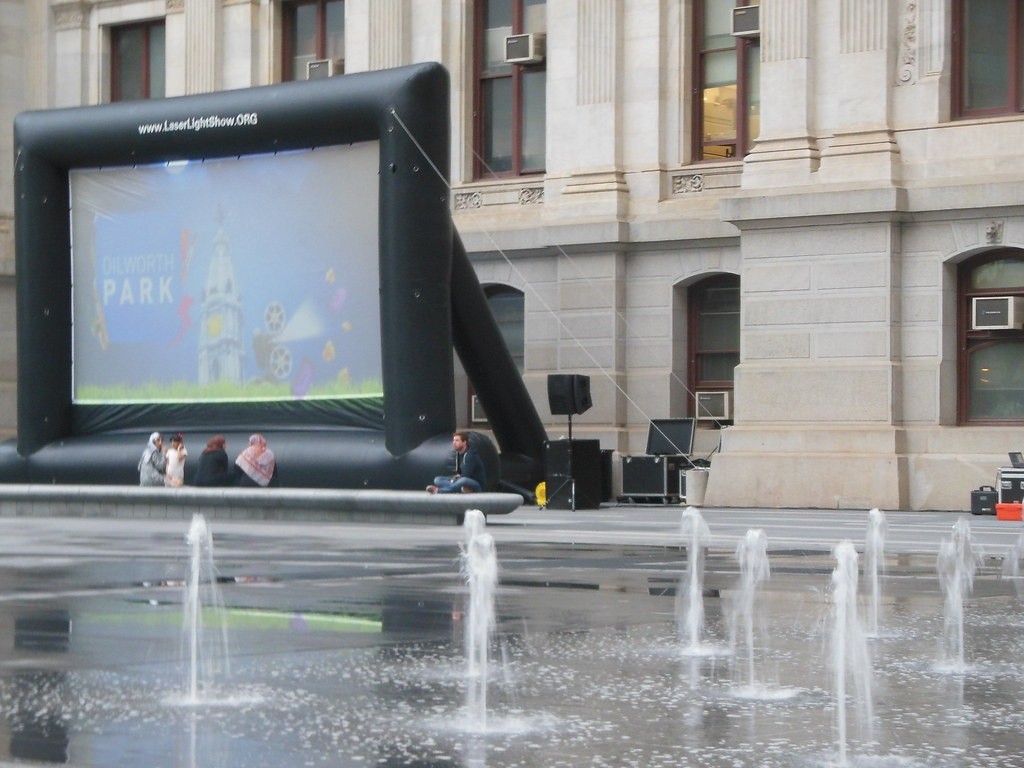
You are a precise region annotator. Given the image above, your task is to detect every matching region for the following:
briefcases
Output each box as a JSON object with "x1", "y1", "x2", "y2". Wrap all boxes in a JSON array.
[{"x1": 971, "y1": 486, "x2": 998, "y2": 515}]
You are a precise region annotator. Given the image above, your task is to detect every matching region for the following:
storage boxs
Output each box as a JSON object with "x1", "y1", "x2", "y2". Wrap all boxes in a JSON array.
[
  {"x1": 619, "y1": 454, "x2": 712, "y2": 505},
  {"x1": 995, "y1": 466, "x2": 1024, "y2": 519}
]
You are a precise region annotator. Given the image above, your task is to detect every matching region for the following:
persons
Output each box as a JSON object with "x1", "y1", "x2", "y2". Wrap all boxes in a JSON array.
[
  {"x1": 234, "y1": 435, "x2": 278, "y2": 488},
  {"x1": 137, "y1": 433, "x2": 188, "y2": 488},
  {"x1": 193, "y1": 435, "x2": 230, "y2": 487},
  {"x1": 426, "y1": 432, "x2": 486, "y2": 494}
]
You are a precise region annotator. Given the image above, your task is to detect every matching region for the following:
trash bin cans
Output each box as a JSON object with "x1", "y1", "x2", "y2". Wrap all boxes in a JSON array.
[{"x1": 685, "y1": 469, "x2": 709, "y2": 507}]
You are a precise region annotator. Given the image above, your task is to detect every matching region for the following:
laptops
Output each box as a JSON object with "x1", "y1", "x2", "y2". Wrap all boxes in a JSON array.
[{"x1": 1009, "y1": 452, "x2": 1024, "y2": 468}]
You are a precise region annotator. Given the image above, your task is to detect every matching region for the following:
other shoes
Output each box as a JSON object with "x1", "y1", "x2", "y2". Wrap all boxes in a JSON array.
[
  {"x1": 426, "y1": 485, "x2": 438, "y2": 494},
  {"x1": 461, "y1": 486, "x2": 473, "y2": 493}
]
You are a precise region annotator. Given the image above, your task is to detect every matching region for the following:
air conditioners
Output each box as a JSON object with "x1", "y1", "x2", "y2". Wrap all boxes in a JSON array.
[
  {"x1": 695, "y1": 391, "x2": 734, "y2": 420},
  {"x1": 971, "y1": 296, "x2": 1024, "y2": 330},
  {"x1": 306, "y1": 58, "x2": 344, "y2": 81},
  {"x1": 504, "y1": 33, "x2": 546, "y2": 65},
  {"x1": 471, "y1": 394, "x2": 490, "y2": 424},
  {"x1": 730, "y1": 5, "x2": 761, "y2": 39}
]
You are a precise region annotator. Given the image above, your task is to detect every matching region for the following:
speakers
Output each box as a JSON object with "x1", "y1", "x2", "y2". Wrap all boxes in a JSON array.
[
  {"x1": 548, "y1": 374, "x2": 593, "y2": 415},
  {"x1": 543, "y1": 438, "x2": 602, "y2": 509}
]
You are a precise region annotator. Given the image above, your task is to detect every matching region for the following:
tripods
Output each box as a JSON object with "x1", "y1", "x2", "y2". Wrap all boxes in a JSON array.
[{"x1": 540, "y1": 415, "x2": 600, "y2": 513}]
